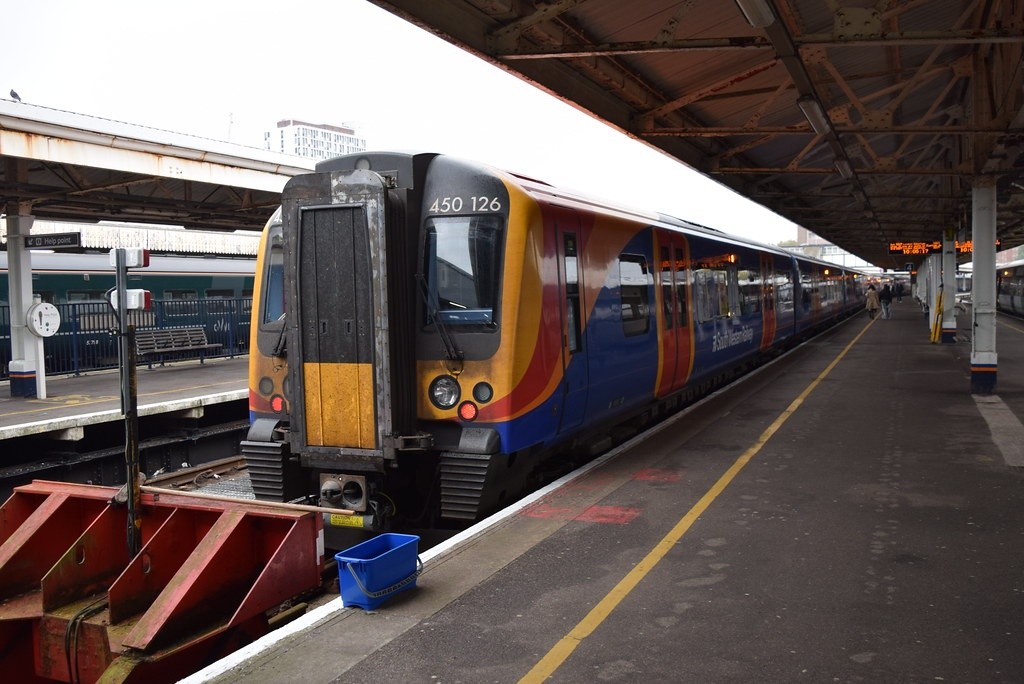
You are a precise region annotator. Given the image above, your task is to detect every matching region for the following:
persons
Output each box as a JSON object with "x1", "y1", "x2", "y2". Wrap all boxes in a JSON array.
[
  {"x1": 801, "y1": 287, "x2": 822, "y2": 312},
  {"x1": 864, "y1": 285, "x2": 880, "y2": 320},
  {"x1": 878, "y1": 284, "x2": 904, "y2": 321}
]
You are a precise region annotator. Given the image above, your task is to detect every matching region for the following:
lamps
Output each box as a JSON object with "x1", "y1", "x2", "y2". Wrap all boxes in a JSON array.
[
  {"x1": 797, "y1": 94, "x2": 832, "y2": 136},
  {"x1": 735, "y1": 0, "x2": 776, "y2": 29},
  {"x1": 853, "y1": 189, "x2": 888, "y2": 246},
  {"x1": 833, "y1": 156, "x2": 854, "y2": 179}
]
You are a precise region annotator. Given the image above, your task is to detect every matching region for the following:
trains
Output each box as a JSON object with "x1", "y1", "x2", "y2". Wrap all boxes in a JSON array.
[
  {"x1": 241, "y1": 149, "x2": 892, "y2": 528},
  {"x1": 956, "y1": 259, "x2": 1024, "y2": 318},
  {"x1": 0, "y1": 254, "x2": 256, "y2": 381}
]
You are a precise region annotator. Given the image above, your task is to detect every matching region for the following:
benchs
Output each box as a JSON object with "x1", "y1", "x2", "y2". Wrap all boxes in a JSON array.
[{"x1": 134, "y1": 328, "x2": 223, "y2": 371}]
